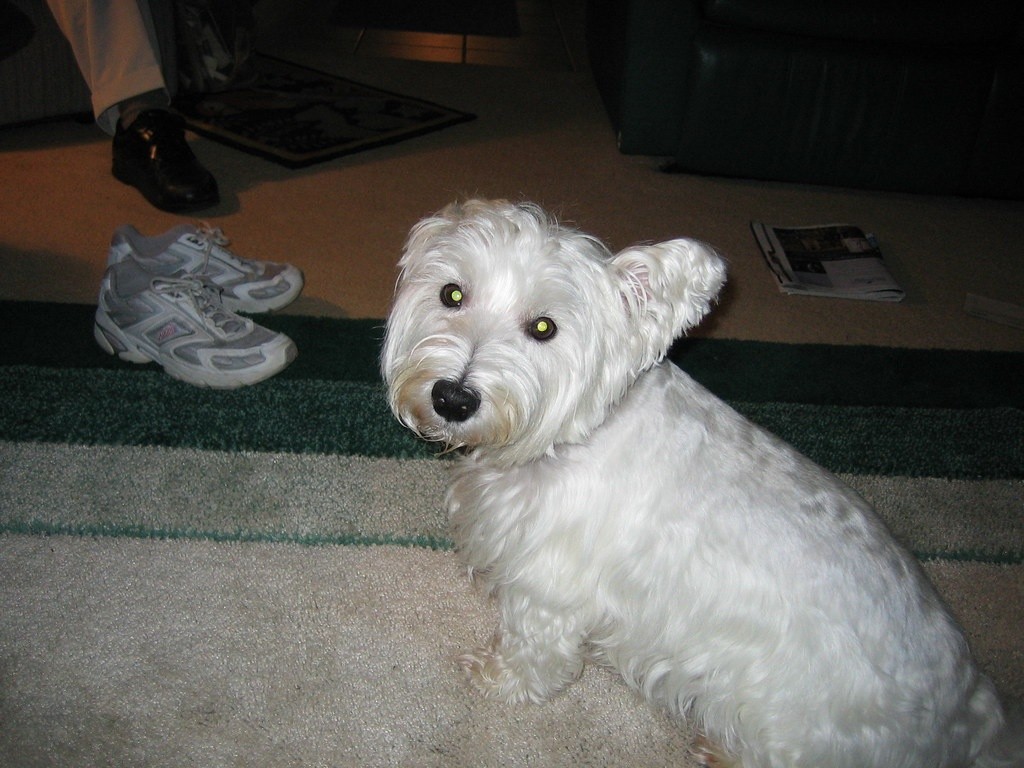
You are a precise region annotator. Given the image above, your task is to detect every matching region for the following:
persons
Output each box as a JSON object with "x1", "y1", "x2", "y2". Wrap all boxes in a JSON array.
[{"x1": 47, "y1": 0, "x2": 220, "y2": 213}]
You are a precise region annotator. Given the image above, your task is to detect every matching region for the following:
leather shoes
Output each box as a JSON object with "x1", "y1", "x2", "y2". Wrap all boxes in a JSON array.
[{"x1": 111, "y1": 108, "x2": 219, "y2": 214}]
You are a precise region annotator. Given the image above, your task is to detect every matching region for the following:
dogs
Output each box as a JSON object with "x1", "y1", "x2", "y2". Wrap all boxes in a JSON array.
[{"x1": 381, "y1": 196, "x2": 1011, "y2": 768}]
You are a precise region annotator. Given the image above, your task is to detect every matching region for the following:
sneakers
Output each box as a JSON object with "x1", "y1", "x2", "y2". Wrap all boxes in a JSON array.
[
  {"x1": 94, "y1": 260, "x2": 298, "y2": 390},
  {"x1": 106, "y1": 223, "x2": 305, "y2": 313}
]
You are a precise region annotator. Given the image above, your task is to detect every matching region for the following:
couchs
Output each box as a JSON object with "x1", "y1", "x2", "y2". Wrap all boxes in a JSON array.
[{"x1": 583, "y1": 0, "x2": 1024, "y2": 201}]
